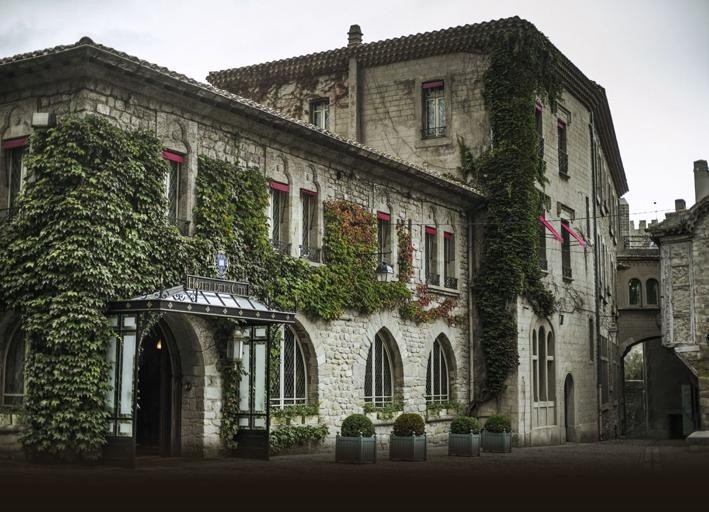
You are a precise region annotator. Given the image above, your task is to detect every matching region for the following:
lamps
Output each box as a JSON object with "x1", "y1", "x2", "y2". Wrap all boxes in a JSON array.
[
  {"x1": 376, "y1": 262, "x2": 394, "y2": 274},
  {"x1": 226, "y1": 329, "x2": 244, "y2": 362}
]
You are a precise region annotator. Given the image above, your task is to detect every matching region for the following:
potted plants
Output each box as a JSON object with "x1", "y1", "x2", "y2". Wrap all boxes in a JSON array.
[
  {"x1": 335, "y1": 415, "x2": 377, "y2": 464},
  {"x1": 448, "y1": 416, "x2": 481, "y2": 457},
  {"x1": 389, "y1": 413, "x2": 426, "y2": 461},
  {"x1": 483, "y1": 416, "x2": 512, "y2": 453}
]
